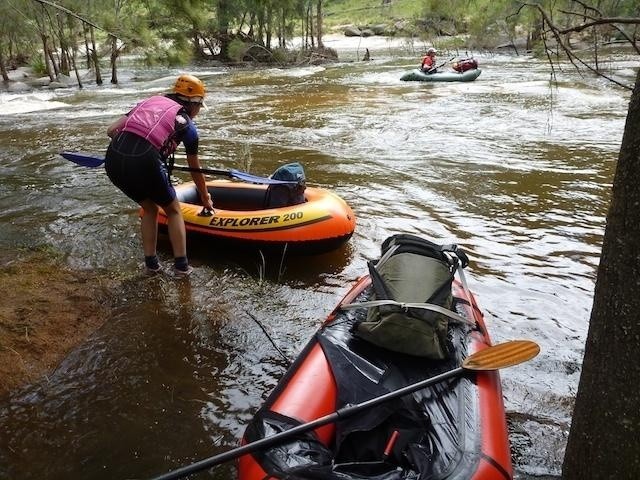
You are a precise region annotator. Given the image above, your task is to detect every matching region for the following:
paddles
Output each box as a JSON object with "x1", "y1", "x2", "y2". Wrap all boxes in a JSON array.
[
  {"x1": 154, "y1": 339, "x2": 541, "y2": 480},
  {"x1": 55, "y1": 151, "x2": 300, "y2": 186},
  {"x1": 427, "y1": 55, "x2": 457, "y2": 72}
]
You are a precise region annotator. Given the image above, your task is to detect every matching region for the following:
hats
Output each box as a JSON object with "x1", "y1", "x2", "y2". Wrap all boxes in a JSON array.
[{"x1": 428, "y1": 48, "x2": 436, "y2": 55}]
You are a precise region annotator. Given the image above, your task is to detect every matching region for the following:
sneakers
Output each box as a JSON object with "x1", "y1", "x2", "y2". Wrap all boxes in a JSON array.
[{"x1": 142, "y1": 263, "x2": 193, "y2": 280}]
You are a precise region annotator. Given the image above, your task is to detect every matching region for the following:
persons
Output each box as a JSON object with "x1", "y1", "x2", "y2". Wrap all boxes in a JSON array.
[
  {"x1": 106, "y1": 74, "x2": 216, "y2": 278},
  {"x1": 420, "y1": 48, "x2": 439, "y2": 75}
]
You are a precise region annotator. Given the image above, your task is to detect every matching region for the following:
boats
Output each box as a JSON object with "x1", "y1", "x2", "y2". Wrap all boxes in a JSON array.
[
  {"x1": 138, "y1": 163, "x2": 356, "y2": 258},
  {"x1": 400, "y1": 67, "x2": 482, "y2": 80},
  {"x1": 237, "y1": 232, "x2": 512, "y2": 480}
]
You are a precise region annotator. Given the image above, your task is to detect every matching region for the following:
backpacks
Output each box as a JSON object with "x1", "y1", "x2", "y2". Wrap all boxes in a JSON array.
[
  {"x1": 351, "y1": 232, "x2": 457, "y2": 363},
  {"x1": 452, "y1": 58, "x2": 478, "y2": 71},
  {"x1": 264, "y1": 163, "x2": 306, "y2": 209}
]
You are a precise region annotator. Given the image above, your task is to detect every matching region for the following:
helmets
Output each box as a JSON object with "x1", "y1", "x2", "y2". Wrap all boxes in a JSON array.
[{"x1": 174, "y1": 74, "x2": 205, "y2": 98}]
